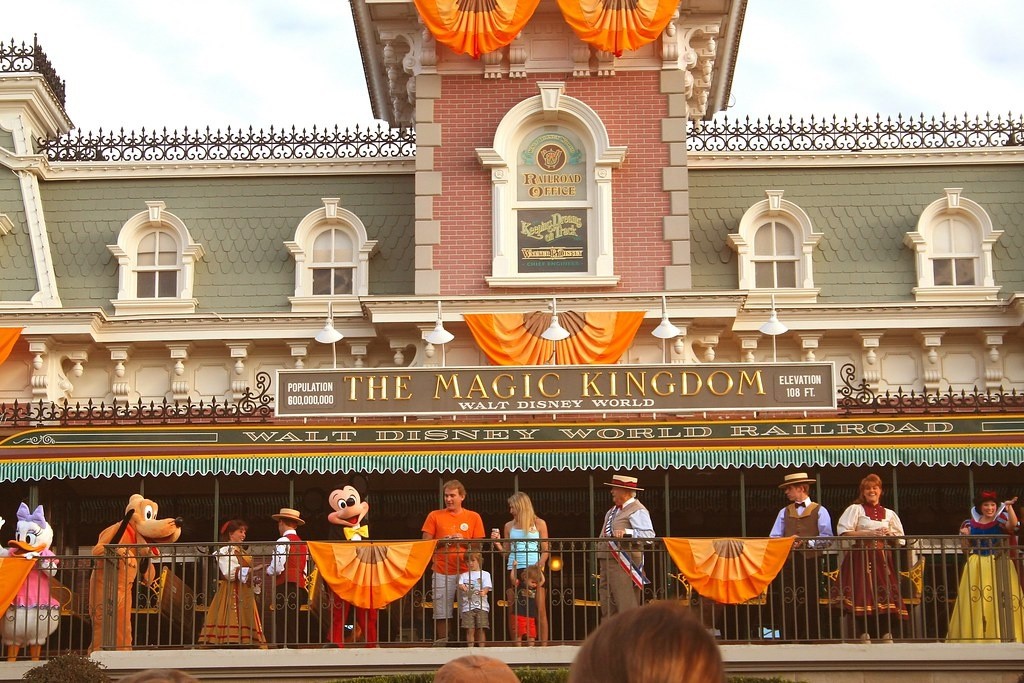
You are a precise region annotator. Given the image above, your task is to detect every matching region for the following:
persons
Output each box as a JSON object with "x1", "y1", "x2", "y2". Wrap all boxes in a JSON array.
[
  {"x1": 266, "y1": 508, "x2": 310, "y2": 649},
  {"x1": 510, "y1": 560, "x2": 542, "y2": 647},
  {"x1": 832, "y1": 474, "x2": 906, "y2": 644},
  {"x1": 422, "y1": 479, "x2": 485, "y2": 647},
  {"x1": 596, "y1": 474, "x2": 655, "y2": 626},
  {"x1": 491, "y1": 492, "x2": 548, "y2": 647},
  {"x1": 434, "y1": 655, "x2": 521, "y2": 683},
  {"x1": 198, "y1": 519, "x2": 271, "y2": 650},
  {"x1": 458, "y1": 548, "x2": 492, "y2": 647},
  {"x1": 567, "y1": 601, "x2": 725, "y2": 683},
  {"x1": 21, "y1": 649, "x2": 207, "y2": 683},
  {"x1": 945, "y1": 491, "x2": 1024, "y2": 644},
  {"x1": 769, "y1": 472, "x2": 833, "y2": 644}
]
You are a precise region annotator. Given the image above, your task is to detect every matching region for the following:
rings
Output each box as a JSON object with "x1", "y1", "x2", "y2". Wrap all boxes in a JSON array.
[{"x1": 618, "y1": 534, "x2": 621, "y2": 535}]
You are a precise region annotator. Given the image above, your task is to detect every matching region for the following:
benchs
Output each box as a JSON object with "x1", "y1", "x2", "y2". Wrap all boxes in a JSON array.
[
  {"x1": 50, "y1": 575, "x2": 92, "y2": 627},
  {"x1": 131, "y1": 567, "x2": 194, "y2": 632},
  {"x1": 270, "y1": 560, "x2": 331, "y2": 642}
]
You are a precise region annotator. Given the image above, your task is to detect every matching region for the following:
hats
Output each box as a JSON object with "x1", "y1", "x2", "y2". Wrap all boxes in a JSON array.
[
  {"x1": 778, "y1": 472, "x2": 817, "y2": 489},
  {"x1": 272, "y1": 508, "x2": 305, "y2": 526},
  {"x1": 603, "y1": 475, "x2": 644, "y2": 491},
  {"x1": 221, "y1": 521, "x2": 231, "y2": 535}
]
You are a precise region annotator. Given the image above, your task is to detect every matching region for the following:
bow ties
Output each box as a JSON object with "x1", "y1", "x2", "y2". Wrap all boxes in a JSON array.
[
  {"x1": 615, "y1": 505, "x2": 623, "y2": 511},
  {"x1": 795, "y1": 502, "x2": 806, "y2": 508}
]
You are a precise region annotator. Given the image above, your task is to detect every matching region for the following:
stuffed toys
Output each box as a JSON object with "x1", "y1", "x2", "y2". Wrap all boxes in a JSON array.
[
  {"x1": 87, "y1": 494, "x2": 184, "y2": 658},
  {"x1": 0, "y1": 502, "x2": 61, "y2": 662},
  {"x1": 303, "y1": 473, "x2": 380, "y2": 649}
]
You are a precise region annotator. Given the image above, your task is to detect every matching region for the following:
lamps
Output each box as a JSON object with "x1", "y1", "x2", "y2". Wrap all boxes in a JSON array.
[
  {"x1": 425, "y1": 301, "x2": 455, "y2": 367},
  {"x1": 542, "y1": 298, "x2": 570, "y2": 365},
  {"x1": 315, "y1": 301, "x2": 343, "y2": 370},
  {"x1": 650, "y1": 294, "x2": 682, "y2": 363},
  {"x1": 758, "y1": 295, "x2": 789, "y2": 363}
]
